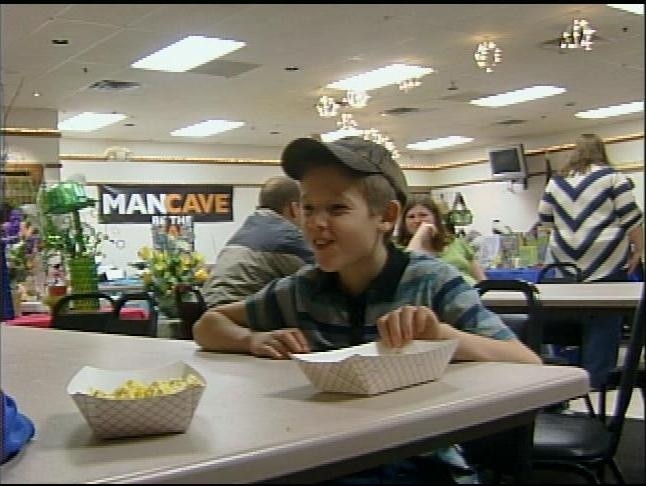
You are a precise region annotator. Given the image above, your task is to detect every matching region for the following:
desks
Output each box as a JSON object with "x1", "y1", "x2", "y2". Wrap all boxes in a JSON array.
[
  {"x1": 0, "y1": 326, "x2": 591, "y2": 486},
  {"x1": 478, "y1": 279, "x2": 645, "y2": 305}
]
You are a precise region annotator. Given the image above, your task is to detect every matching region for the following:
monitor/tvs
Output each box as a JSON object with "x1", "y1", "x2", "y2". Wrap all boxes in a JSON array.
[{"x1": 488, "y1": 144, "x2": 528, "y2": 180}]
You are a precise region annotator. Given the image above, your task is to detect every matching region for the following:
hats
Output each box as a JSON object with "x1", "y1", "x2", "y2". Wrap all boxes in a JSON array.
[{"x1": 281, "y1": 135, "x2": 409, "y2": 204}]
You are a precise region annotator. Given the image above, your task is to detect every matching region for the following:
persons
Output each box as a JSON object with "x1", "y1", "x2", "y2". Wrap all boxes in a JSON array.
[
  {"x1": 201, "y1": 176, "x2": 315, "y2": 308},
  {"x1": 538, "y1": 132, "x2": 646, "y2": 391},
  {"x1": 191, "y1": 135, "x2": 547, "y2": 366},
  {"x1": 392, "y1": 194, "x2": 489, "y2": 290}
]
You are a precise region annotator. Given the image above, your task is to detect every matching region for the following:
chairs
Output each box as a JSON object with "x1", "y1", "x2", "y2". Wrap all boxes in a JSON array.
[
  {"x1": 117, "y1": 292, "x2": 158, "y2": 336},
  {"x1": 51, "y1": 292, "x2": 117, "y2": 333},
  {"x1": 176, "y1": 286, "x2": 207, "y2": 340},
  {"x1": 533, "y1": 282, "x2": 646, "y2": 485}
]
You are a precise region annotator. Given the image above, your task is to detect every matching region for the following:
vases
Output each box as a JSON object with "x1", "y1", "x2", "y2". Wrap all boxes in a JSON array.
[{"x1": 160, "y1": 294, "x2": 179, "y2": 317}]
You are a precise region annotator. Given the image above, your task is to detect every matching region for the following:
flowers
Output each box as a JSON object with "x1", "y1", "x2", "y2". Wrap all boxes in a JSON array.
[{"x1": 139, "y1": 233, "x2": 213, "y2": 298}]
